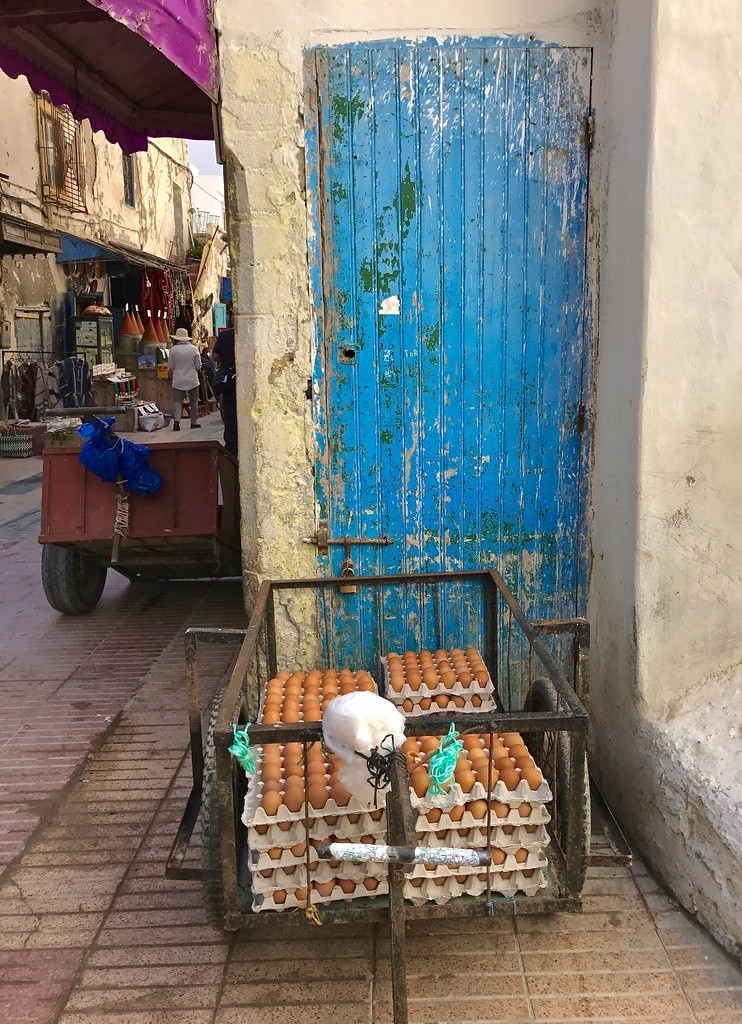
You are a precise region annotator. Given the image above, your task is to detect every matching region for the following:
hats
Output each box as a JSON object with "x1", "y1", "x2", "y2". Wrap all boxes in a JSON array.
[{"x1": 170, "y1": 328, "x2": 192, "y2": 341}]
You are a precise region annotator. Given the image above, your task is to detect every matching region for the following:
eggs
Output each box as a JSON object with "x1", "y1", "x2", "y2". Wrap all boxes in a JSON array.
[
  {"x1": 254, "y1": 668, "x2": 381, "y2": 902},
  {"x1": 387, "y1": 647, "x2": 542, "y2": 887}
]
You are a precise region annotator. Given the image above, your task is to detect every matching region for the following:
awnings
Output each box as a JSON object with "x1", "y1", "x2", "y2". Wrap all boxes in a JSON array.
[{"x1": 108, "y1": 241, "x2": 190, "y2": 273}]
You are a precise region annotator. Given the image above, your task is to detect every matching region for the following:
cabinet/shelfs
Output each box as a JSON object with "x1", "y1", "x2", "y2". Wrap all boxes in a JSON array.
[
  {"x1": 69, "y1": 316, "x2": 114, "y2": 373},
  {"x1": 93, "y1": 378, "x2": 157, "y2": 431}
]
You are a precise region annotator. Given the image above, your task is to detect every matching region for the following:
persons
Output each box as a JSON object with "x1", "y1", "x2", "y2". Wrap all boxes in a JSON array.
[
  {"x1": 211, "y1": 308, "x2": 238, "y2": 458},
  {"x1": 168, "y1": 328, "x2": 202, "y2": 432},
  {"x1": 202, "y1": 347, "x2": 220, "y2": 410}
]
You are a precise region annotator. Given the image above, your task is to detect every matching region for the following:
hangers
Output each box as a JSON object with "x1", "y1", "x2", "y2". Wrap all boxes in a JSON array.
[{"x1": 10, "y1": 351, "x2": 81, "y2": 364}]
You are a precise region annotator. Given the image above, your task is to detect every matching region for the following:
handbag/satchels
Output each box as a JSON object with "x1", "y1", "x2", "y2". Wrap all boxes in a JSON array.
[
  {"x1": 212, "y1": 369, "x2": 233, "y2": 392},
  {"x1": 0, "y1": 427, "x2": 33, "y2": 458}
]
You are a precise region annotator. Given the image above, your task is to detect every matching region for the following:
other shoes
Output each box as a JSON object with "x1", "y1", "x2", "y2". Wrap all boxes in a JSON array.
[
  {"x1": 174, "y1": 421, "x2": 180, "y2": 430},
  {"x1": 191, "y1": 424, "x2": 201, "y2": 428}
]
